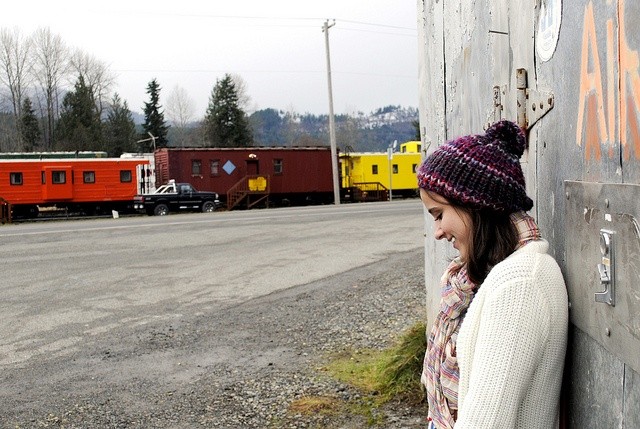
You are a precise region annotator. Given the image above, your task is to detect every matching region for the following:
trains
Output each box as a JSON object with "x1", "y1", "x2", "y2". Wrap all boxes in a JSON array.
[{"x1": 1, "y1": 141, "x2": 423, "y2": 222}]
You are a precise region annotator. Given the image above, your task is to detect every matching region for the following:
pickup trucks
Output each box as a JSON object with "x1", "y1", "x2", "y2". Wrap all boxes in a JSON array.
[{"x1": 135, "y1": 179, "x2": 221, "y2": 215}]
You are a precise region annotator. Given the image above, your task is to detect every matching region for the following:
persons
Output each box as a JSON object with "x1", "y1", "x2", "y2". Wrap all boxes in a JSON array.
[{"x1": 414, "y1": 119, "x2": 570, "y2": 429}]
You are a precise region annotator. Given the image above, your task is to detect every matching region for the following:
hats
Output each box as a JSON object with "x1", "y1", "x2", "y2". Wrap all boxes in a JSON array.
[{"x1": 416, "y1": 120, "x2": 531, "y2": 212}]
